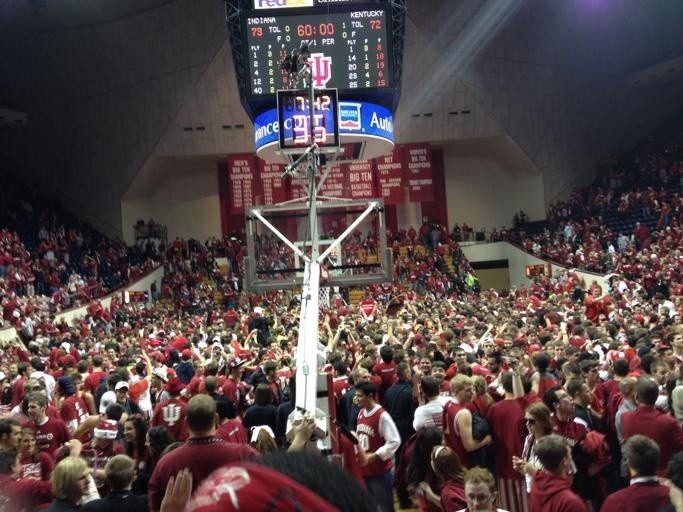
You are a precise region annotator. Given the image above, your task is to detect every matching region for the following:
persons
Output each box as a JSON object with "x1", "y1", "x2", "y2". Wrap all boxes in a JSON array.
[{"x1": 4, "y1": 140, "x2": 683, "y2": 512}]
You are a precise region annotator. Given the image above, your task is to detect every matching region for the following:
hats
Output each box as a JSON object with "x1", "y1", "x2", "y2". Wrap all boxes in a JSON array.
[
  {"x1": 99, "y1": 391, "x2": 117, "y2": 414},
  {"x1": 638, "y1": 344, "x2": 655, "y2": 358},
  {"x1": 62, "y1": 342, "x2": 70, "y2": 354},
  {"x1": 511, "y1": 338, "x2": 527, "y2": 347},
  {"x1": 58, "y1": 375, "x2": 77, "y2": 395},
  {"x1": 152, "y1": 367, "x2": 185, "y2": 393},
  {"x1": 114, "y1": 381, "x2": 130, "y2": 392},
  {"x1": 413, "y1": 334, "x2": 425, "y2": 347},
  {"x1": 481, "y1": 338, "x2": 495, "y2": 347},
  {"x1": 228, "y1": 357, "x2": 247, "y2": 366},
  {"x1": 182, "y1": 349, "x2": 193, "y2": 356},
  {"x1": 93, "y1": 418, "x2": 119, "y2": 439},
  {"x1": 148, "y1": 340, "x2": 162, "y2": 351},
  {"x1": 148, "y1": 351, "x2": 166, "y2": 363}
]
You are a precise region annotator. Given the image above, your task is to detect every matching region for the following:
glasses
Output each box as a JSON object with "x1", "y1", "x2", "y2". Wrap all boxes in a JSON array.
[
  {"x1": 557, "y1": 393, "x2": 569, "y2": 401},
  {"x1": 522, "y1": 417, "x2": 539, "y2": 424}
]
